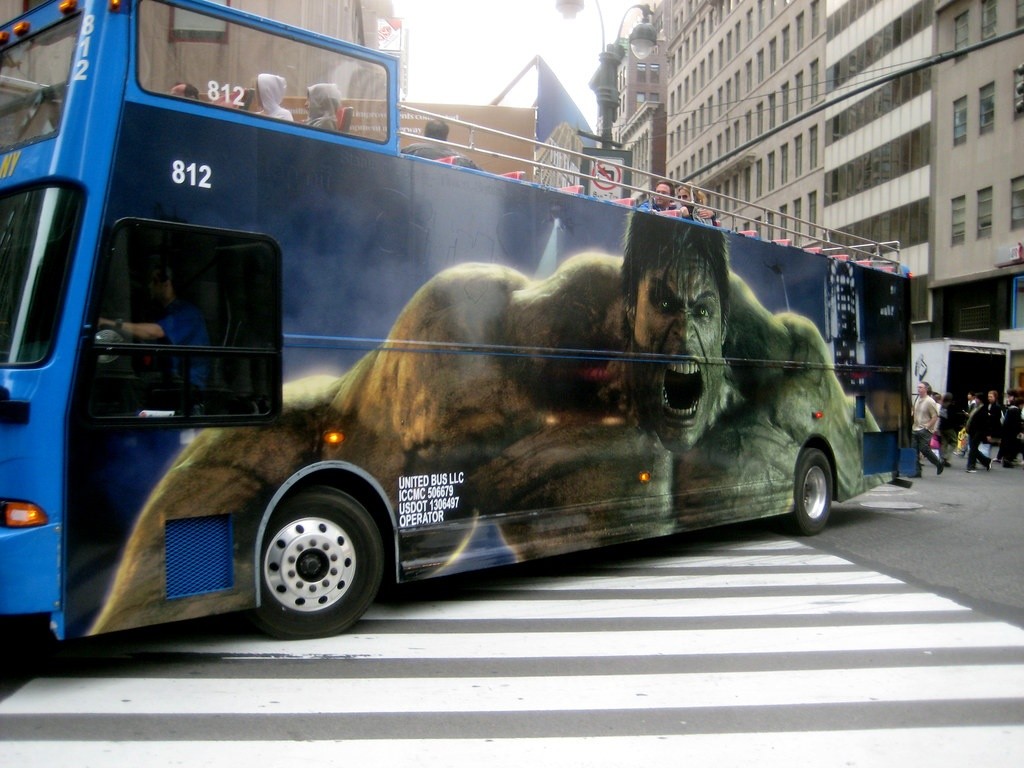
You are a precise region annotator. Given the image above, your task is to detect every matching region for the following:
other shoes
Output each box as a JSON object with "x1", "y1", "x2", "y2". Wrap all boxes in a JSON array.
[
  {"x1": 940, "y1": 461, "x2": 950, "y2": 467},
  {"x1": 909, "y1": 474, "x2": 921, "y2": 478},
  {"x1": 986, "y1": 457, "x2": 992, "y2": 472},
  {"x1": 965, "y1": 469, "x2": 977, "y2": 473},
  {"x1": 937, "y1": 460, "x2": 944, "y2": 474},
  {"x1": 953, "y1": 452, "x2": 964, "y2": 458},
  {"x1": 993, "y1": 458, "x2": 1002, "y2": 463}
]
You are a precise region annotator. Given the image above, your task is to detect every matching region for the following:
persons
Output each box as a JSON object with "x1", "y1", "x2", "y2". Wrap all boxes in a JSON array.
[
  {"x1": 84, "y1": 211, "x2": 882, "y2": 633},
  {"x1": 165, "y1": 71, "x2": 716, "y2": 227},
  {"x1": 94, "y1": 262, "x2": 215, "y2": 415},
  {"x1": 910, "y1": 381, "x2": 1024, "y2": 476}
]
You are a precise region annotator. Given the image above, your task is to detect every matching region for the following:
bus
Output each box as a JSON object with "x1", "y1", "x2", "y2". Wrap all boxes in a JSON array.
[{"x1": 1, "y1": 0, "x2": 925, "y2": 659}]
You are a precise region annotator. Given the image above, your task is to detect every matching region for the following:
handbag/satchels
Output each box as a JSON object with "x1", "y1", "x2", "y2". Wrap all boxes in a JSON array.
[
  {"x1": 957, "y1": 426, "x2": 967, "y2": 450},
  {"x1": 930, "y1": 432, "x2": 940, "y2": 449},
  {"x1": 978, "y1": 440, "x2": 991, "y2": 458}
]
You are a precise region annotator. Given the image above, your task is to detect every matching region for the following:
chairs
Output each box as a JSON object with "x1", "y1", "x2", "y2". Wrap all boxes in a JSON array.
[
  {"x1": 773, "y1": 239, "x2": 792, "y2": 247},
  {"x1": 559, "y1": 185, "x2": 585, "y2": 196},
  {"x1": 803, "y1": 247, "x2": 822, "y2": 255},
  {"x1": 211, "y1": 89, "x2": 256, "y2": 110},
  {"x1": 614, "y1": 198, "x2": 638, "y2": 207},
  {"x1": 856, "y1": 260, "x2": 873, "y2": 267},
  {"x1": 828, "y1": 254, "x2": 850, "y2": 261},
  {"x1": 435, "y1": 156, "x2": 464, "y2": 168},
  {"x1": 505, "y1": 171, "x2": 528, "y2": 182},
  {"x1": 335, "y1": 105, "x2": 356, "y2": 134},
  {"x1": 662, "y1": 210, "x2": 683, "y2": 217},
  {"x1": 877, "y1": 266, "x2": 896, "y2": 273},
  {"x1": 738, "y1": 229, "x2": 758, "y2": 239}
]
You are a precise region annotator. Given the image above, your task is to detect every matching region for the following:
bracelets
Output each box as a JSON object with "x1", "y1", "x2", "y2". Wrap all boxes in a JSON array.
[{"x1": 111, "y1": 318, "x2": 123, "y2": 330}]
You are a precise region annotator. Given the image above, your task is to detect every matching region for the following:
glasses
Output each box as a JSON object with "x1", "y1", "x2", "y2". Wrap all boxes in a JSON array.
[{"x1": 678, "y1": 194, "x2": 689, "y2": 199}]
